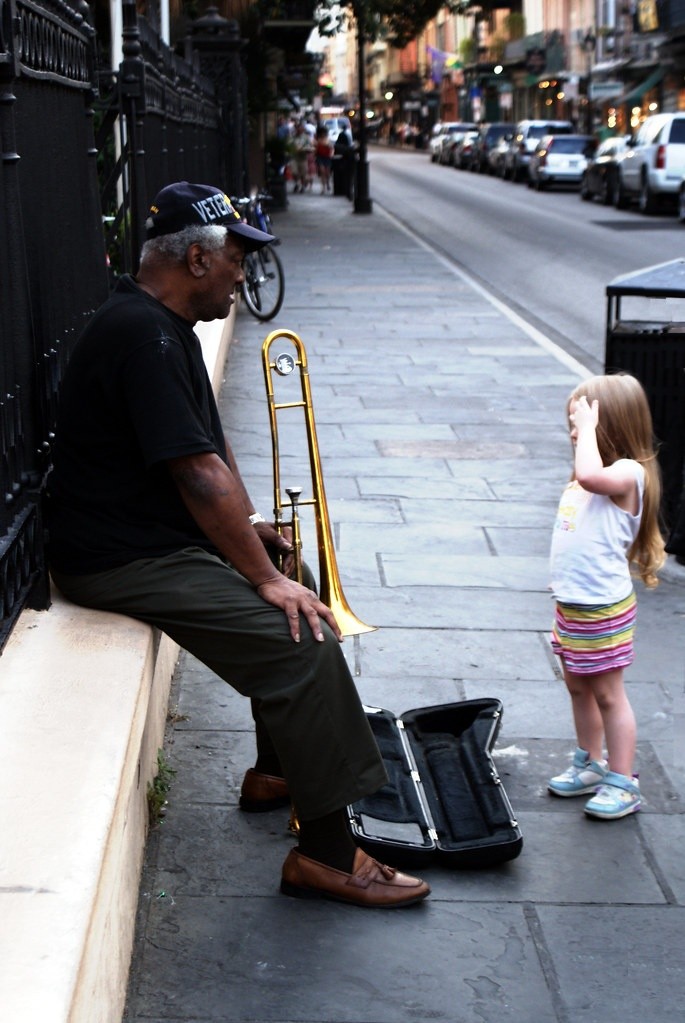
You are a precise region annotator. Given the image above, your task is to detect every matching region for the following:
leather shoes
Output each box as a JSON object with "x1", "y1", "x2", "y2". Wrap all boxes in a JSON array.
[
  {"x1": 280, "y1": 846, "x2": 431, "y2": 908},
  {"x1": 239, "y1": 766, "x2": 288, "y2": 812}
]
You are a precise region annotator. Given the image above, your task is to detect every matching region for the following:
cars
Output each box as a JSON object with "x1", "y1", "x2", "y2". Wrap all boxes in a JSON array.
[
  {"x1": 580, "y1": 136, "x2": 627, "y2": 204},
  {"x1": 528, "y1": 135, "x2": 600, "y2": 192},
  {"x1": 324, "y1": 118, "x2": 352, "y2": 150},
  {"x1": 426, "y1": 121, "x2": 576, "y2": 184}
]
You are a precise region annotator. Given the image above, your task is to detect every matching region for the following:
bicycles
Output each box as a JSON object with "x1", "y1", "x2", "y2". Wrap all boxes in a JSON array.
[{"x1": 228, "y1": 191, "x2": 286, "y2": 321}]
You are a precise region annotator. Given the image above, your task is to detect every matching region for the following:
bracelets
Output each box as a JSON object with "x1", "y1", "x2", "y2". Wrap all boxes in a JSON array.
[{"x1": 248, "y1": 513, "x2": 265, "y2": 526}]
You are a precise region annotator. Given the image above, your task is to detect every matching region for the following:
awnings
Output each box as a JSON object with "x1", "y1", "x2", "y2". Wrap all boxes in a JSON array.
[{"x1": 465, "y1": 64, "x2": 516, "y2": 74}]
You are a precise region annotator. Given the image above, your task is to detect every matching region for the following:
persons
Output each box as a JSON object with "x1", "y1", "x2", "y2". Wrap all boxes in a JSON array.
[
  {"x1": 544, "y1": 371, "x2": 664, "y2": 821},
  {"x1": 393, "y1": 118, "x2": 428, "y2": 149},
  {"x1": 268, "y1": 105, "x2": 353, "y2": 197},
  {"x1": 51, "y1": 180, "x2": 430, "y2": 910}
]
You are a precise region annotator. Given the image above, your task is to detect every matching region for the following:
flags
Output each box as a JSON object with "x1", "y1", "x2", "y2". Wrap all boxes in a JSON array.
[{"x1": 427, "y1": 45, "x2": 459, "y2": 88}]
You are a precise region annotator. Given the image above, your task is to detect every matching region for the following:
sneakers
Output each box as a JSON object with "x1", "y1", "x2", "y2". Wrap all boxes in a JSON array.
[
  {"x1": 548, "y1": 746, "x2": 609, "y2": 796},
  {"x1": 584, "y1": 771, "x2": 641, "y2": 819}
]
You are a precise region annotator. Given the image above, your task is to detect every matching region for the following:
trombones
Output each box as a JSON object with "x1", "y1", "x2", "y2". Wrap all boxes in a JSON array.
[{"x1": 261, "y1": 327, "x2": 380, "y2": 833}]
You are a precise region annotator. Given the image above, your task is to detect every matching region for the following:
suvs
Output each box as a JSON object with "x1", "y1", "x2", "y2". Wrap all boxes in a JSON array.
[{"x1": 612, "y1": 113, "x2": 685, "y2": 216}]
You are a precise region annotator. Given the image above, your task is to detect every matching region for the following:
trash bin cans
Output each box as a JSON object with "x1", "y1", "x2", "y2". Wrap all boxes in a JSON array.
[
  {"x1": 332, "y1": 154, "x2": 349, "y2": 196},
  {"x1": 606, "y1": 259, "x2": 685, "y2": 567}
]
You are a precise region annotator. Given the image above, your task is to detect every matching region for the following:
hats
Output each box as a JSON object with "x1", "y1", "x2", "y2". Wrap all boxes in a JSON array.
[{"x1": 145, "y1": 183, "x2": 276, "y2": 255}]
